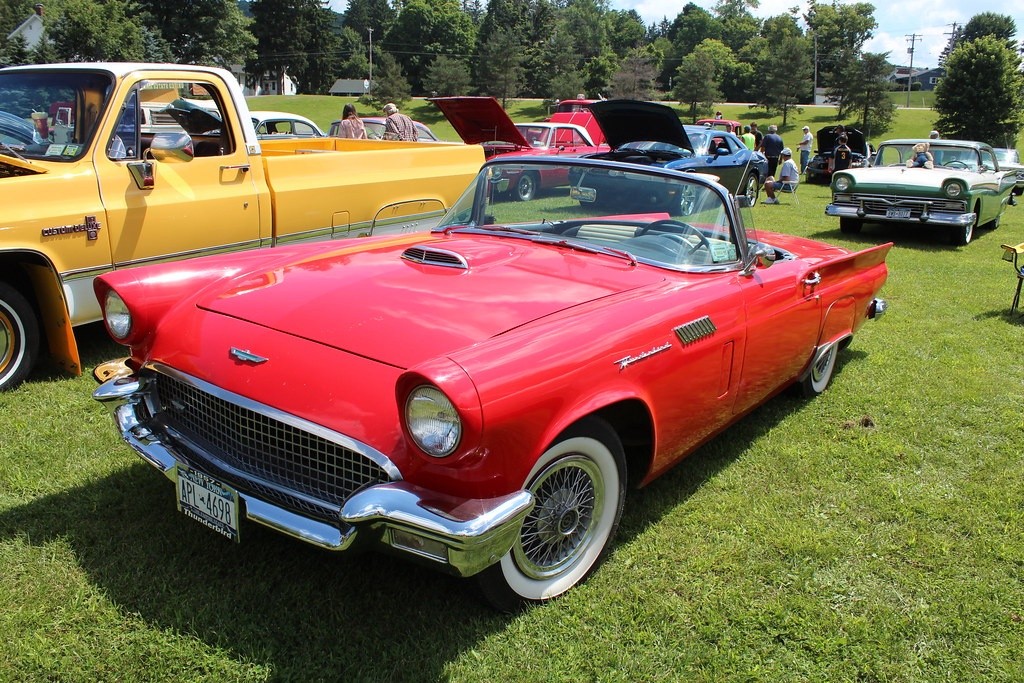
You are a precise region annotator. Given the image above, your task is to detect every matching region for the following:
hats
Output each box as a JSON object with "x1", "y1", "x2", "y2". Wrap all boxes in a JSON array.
[
  {"x1": 383, "y1": 103, "x2": 395, "y2": 111},
  {"x1": 838, "y1": 125, "x2": 844, "y2": 130},
  {"x1": 781, "y1": 147, "x2": 792, "y2": 156},
  {"x1": 802, "y1": 126, "x2": 809, "y2": 130},
  {"x1": 744, "y1": 126, "x2": 751, "y2": 131}
]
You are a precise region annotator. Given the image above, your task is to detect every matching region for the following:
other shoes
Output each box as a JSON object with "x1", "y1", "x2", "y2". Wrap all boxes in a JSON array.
[{"x1": 761, "y1": 197, "x2": 778, "y2": 204}]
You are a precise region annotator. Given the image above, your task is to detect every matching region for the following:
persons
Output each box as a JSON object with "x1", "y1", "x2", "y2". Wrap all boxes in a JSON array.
[
  {"x1": 715, "y1": 111, "x2": 812, "y2": 203},
  {"x1": 381, "y1": 103, "x2": 419, "y2": 142},
  {"x1": 337, "y1": 103, "x2": 368, "y2": 140},
  {"x1": 828, "y1": 124, "x2": 853, "y2": 174},
  {"x1": 926, "y1": 131, "x2": 944, "y2": 166}
]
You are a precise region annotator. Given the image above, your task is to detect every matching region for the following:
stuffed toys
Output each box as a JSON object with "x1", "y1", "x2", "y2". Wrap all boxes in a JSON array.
[{"x1": 906, "y1": 143, "x2": 933, "y2": 168}]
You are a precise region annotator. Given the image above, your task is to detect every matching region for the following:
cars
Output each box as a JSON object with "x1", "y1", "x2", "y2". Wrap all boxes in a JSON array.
[
  {"x1": 92, "y1": 151, "x2": 894, "y2": 602},
  {"x1": 805, "y1": 125, "x2": 884, "y2": 184},
  {"x1": 952, "y1": 147, "x2": 1024, "y2": 196},
  {"x1": 568, "y1": 98, "x2": 768, "y2": 216},
  {"x1": 824, "y1": 137, "x2": 1017, "y2": 245},
  {"x1": 690, "y1": 119, "x2": 746, "y2": 155},
  {"x1": 330, "y1": 117, "x2": 446, "y2": 144},
  {"x1": 422, "y1": 95, "x2": 612, "y2": 202},
  {"x1": 249, "y1": 110, "x2": 327, "y2": 139}
]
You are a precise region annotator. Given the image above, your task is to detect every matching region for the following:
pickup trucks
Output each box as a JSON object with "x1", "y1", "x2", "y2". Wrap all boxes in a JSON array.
[{"x1": 0, "y1": 61, "x2": 495, "y2": 394}]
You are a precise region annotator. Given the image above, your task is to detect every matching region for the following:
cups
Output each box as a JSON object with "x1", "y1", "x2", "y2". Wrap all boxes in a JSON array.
[{"x1": 31, "y1": 112, "x2": 49, "y2": 144}]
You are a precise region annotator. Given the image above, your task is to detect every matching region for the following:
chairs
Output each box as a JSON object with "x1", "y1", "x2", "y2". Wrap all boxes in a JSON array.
[
  {"x1": 772, "y1": 165, "x2": 808, "y2": 205},
  {"x1": 709, "y1": 140, "x2": 717, "y2": 154},
  {"x1": 1000, "y1": 241, "x2": 1024, "y2": 317}
]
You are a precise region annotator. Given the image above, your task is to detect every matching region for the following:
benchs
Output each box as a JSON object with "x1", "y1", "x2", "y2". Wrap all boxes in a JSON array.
[
  {"x1": 105, "y1": 135, "x2": 217, "y2": 158},
  {"x1": 561, "y1": 224, "x2": 737, "y2": 266},
  {"x1": 717, "y1": 142, "x2": 728, "y2": 154},
  {"x1": 549, "y1": 142, "x2": 585, "y2": 150}
]
